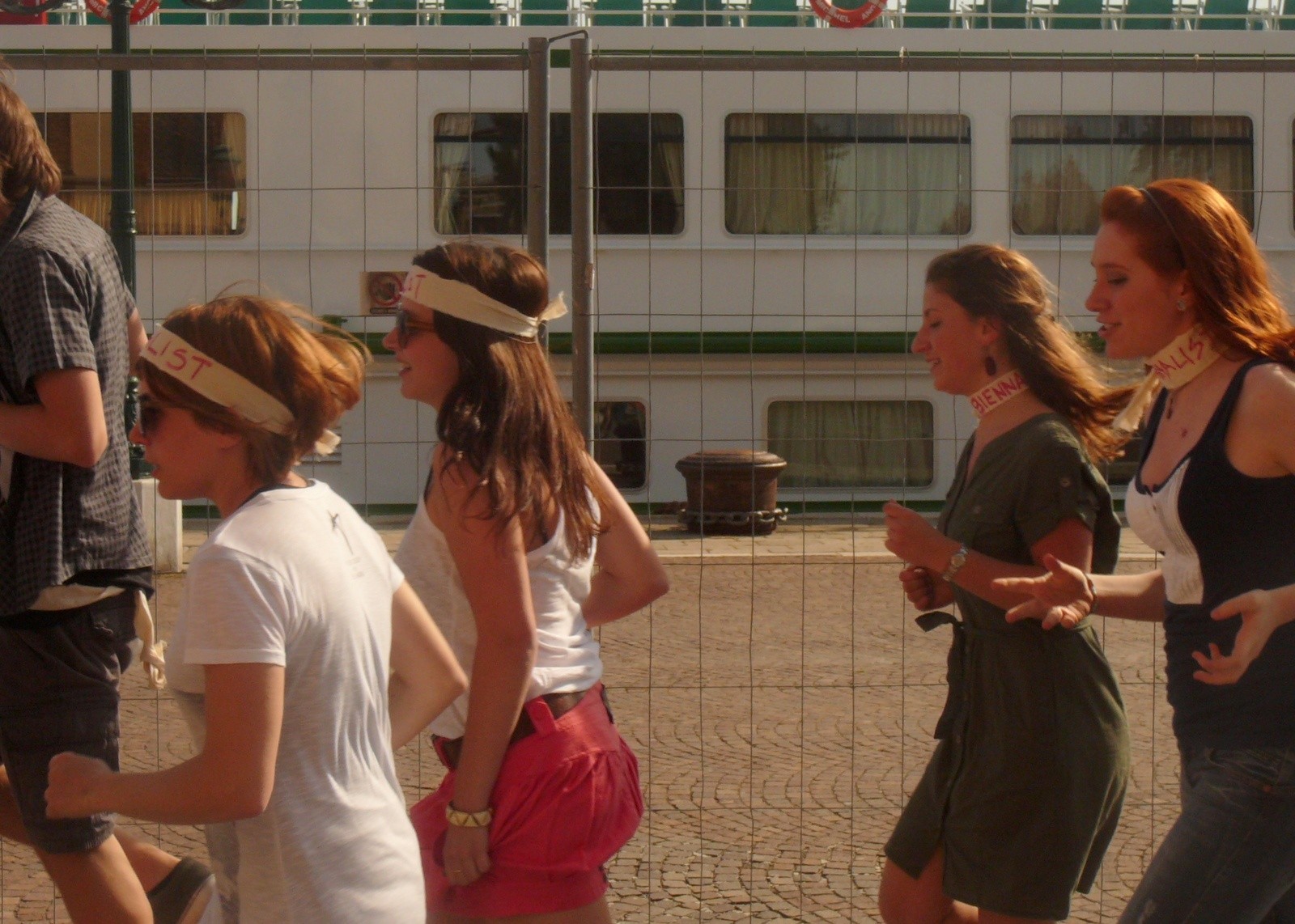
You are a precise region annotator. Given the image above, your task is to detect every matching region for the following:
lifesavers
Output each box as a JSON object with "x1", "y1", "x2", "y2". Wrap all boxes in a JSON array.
[
  {"x1": 84, "y1": 0, "x2": 161, "y2": 25},
  {"x1": 807, "y1": 1, "x2": 887, "y2": 28}
]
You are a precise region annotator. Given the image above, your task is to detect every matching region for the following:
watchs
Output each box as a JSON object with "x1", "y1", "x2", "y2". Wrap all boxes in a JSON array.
[{"x1": 942, "y1": 542, "x2": 969, "y2": 583}]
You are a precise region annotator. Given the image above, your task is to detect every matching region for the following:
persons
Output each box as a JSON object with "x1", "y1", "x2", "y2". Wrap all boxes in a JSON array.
[
  {"x1": 990, "y1": 179, "x2": 1295, "y2": 924},
  {"x1": 877, "y1": 243, "x2": 1137, "y2": 924},
  {"x1": 0, "y1": 85, "x2": 222, "y2": 924},
  {"x1": 378, "y1": 239, "x2": 672, "y2": 922},
  {"x1": 44, "y1": 290, "x2": 470, "y2": 924}
]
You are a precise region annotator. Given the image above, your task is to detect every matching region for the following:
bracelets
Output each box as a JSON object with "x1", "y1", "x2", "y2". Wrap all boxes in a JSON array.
[{"x1": 442, "y1": 802, "x2": 495, "y2": 827}]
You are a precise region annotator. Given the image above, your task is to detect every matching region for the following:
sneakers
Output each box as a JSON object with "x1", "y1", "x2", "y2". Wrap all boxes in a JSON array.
[{"x1": 148, "y1": 856, "x2": 215, "y2": 924}]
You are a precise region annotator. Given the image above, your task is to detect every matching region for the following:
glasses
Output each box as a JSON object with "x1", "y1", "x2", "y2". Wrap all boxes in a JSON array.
[
  {"x1": 395, "y1": 307, "x2": 438, "y2": 344},
  {"x1": 132, "y1": 398, "x2": 189, "y2": 436}
]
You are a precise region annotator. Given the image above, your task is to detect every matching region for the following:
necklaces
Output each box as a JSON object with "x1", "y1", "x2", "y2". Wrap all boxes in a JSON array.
[
  {"x1": 968, "y1": 369, "x2": 1026, "y2": 417},
  {"x1": 1119, "y1": 317, "x2": 1228, "y2": 433}
]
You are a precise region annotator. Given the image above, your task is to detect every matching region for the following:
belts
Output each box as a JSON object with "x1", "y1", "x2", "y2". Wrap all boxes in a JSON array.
[{"x1": 430, "y1": 690, "x2": 589, "y2": 769}]
